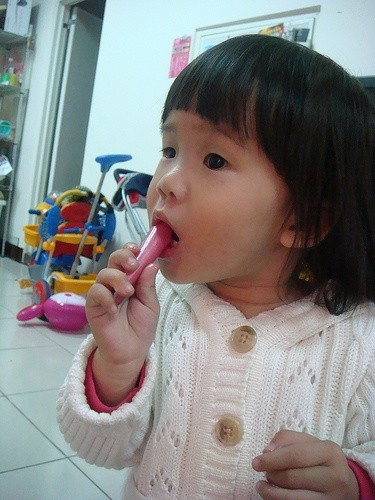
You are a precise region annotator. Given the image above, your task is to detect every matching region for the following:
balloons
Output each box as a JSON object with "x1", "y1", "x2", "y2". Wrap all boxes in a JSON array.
[{"x1": 15, "y1": 291, "x2": 89, "y2": 330}]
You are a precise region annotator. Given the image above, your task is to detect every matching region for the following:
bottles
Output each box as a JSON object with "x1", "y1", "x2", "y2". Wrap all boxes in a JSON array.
[{"x1": 6, "y1": 57, "x2": 14, "y2": 74}]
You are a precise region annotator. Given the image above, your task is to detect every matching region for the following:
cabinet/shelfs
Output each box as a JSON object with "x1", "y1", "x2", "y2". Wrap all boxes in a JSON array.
[{"x1": 0, "y1": 24, "x2": 33, "y2": 256}]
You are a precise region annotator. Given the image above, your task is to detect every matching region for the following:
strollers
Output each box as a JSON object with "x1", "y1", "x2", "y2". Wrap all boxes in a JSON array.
[{"x1": 24, "y1": 153, "x2": 133, "y2": 321}]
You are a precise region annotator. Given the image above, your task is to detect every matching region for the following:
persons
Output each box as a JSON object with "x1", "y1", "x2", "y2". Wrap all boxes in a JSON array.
[{"x1": 61, "y1": 33, "x2": 375, "y2": 500}]
